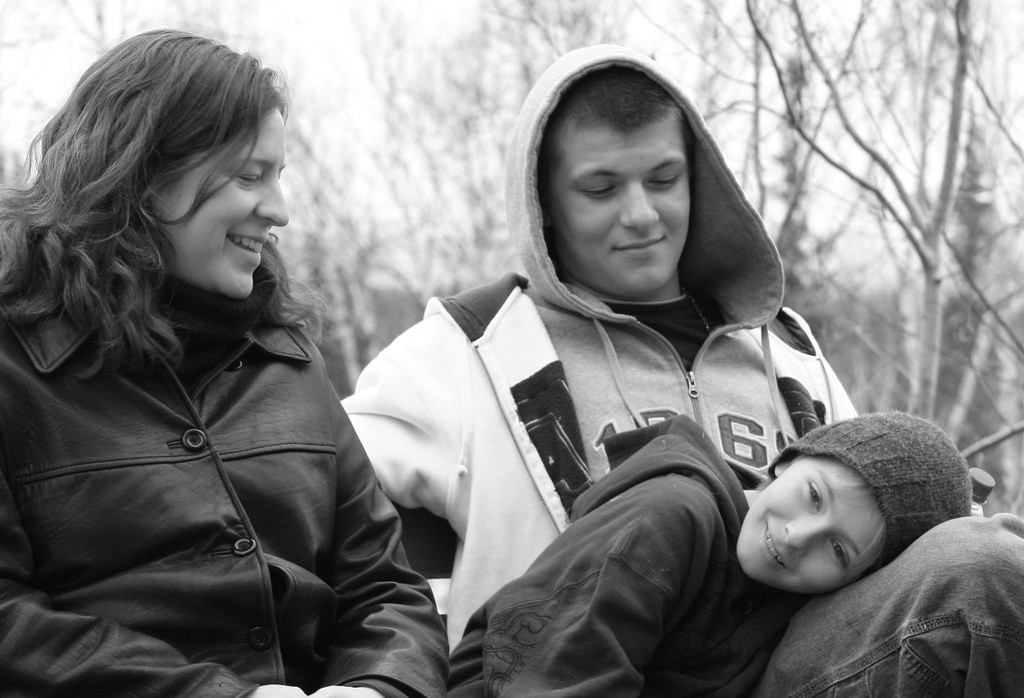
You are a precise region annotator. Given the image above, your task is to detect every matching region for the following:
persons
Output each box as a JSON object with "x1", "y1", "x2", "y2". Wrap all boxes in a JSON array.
[
  {"x1": 0, "y1": 28, "x2": 452, "y2": 698},
  {"x1": 443, "y1": 408, "x2": 974, "y2": 698},
  {"x1": 341, "y1": 41, "x2": 1023, "y2": 698}
]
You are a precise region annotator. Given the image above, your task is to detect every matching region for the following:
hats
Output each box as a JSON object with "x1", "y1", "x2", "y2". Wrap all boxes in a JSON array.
[{"x1": 769, "y1": 410, "x2": 973, "y2": 578}]
